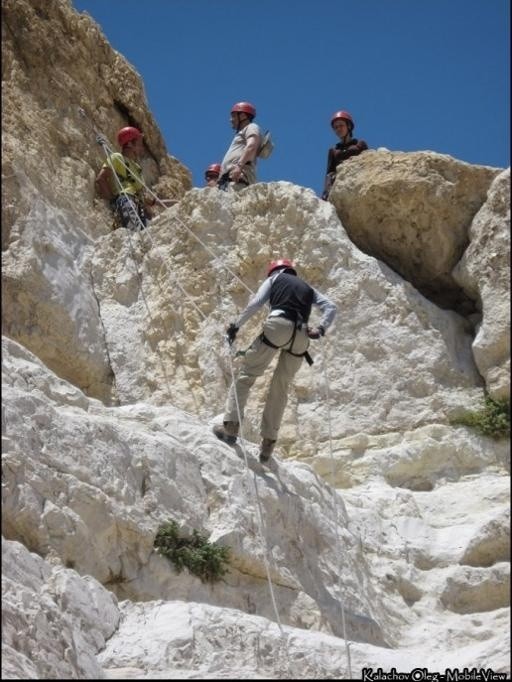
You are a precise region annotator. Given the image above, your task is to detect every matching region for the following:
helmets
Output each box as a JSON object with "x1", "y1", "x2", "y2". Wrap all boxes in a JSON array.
[
  {"x1": 117, "y1": 127, "x2": 144, "y2": 146},
  {"x1": 331, "y1": 111, "x2": 354, "y2": 133},
  {"x1": 230, "y1": 102, "x2": 256, "y2": 118},
  {"x1": 205, "y1": 164, "x2": 221, "y2": 175},
  {"x1": 268, "y1": 259, "x2": 297, "y2": 277}
]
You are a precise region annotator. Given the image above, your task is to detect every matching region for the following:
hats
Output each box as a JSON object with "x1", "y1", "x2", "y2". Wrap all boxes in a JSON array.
[{"x1": 257, "y1": 130, "x2": 274, "y2": 160}]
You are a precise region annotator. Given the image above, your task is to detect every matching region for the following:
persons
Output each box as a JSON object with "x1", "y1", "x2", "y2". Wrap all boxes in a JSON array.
[
  {"x1": 212, "y1": 258, "x2": 339, "y2": 465},
  {"x1": 216, "y1": 101, "x2": 262, "y2": 195},
  {"x1": 94, "y1": 126, "x2": 159, "y2": 233},
  {"x1": 319, "y1": 111, "x2": 369, "y2": 200},
  {"x1": 204, "y1": 164, "x2": 220, "y2": 188}
]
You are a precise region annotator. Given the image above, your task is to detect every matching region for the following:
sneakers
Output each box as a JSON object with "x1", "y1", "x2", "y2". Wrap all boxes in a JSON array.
[
  {"x1": 259, "y1": 439, "x2": 276, "y2": 462},
  {"x1": 213, "y1": 421, "x2": 238, "y2": 443}
]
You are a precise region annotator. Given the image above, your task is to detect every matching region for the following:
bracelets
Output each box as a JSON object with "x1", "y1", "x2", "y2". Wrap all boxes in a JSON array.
[{"x1": 235, "y1": 164, "x2": 243, "y2": 171}]
